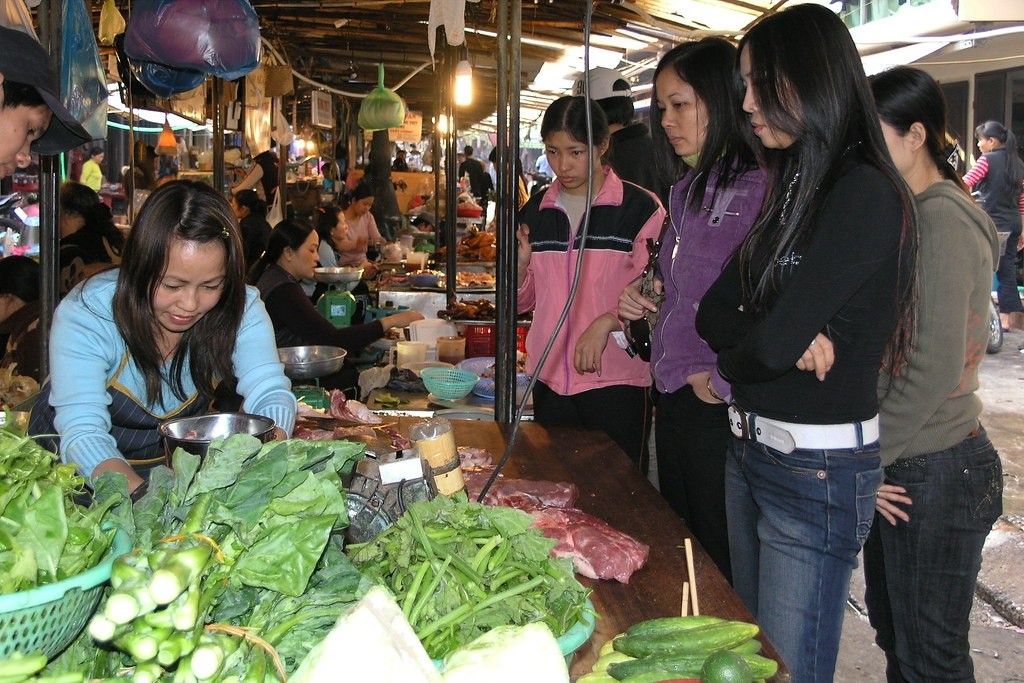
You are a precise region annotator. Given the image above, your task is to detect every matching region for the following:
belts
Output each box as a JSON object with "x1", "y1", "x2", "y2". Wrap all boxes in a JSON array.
[{"x1": 728, "y1": 402, "x2": 881, "y2": 454}]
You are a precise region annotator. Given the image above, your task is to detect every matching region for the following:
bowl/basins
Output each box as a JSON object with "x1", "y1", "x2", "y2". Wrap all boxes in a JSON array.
[
  {"x1": 458, "y1": 209, "x2": 483, "y2": 218},
  {"x1": 315, "y1": 267, "x2": 365, "y2": 282},
  {"x1": 156, "y1": 413, "x2": 276, "y2": 464},
  {"x1": 278, "y1": 345, "x2": 347, "y2": 379}
]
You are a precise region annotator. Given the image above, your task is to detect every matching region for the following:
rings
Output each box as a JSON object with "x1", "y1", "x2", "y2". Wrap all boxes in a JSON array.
[{"x1": 809, "y1": 338, "x2": 816, "y2": 346}]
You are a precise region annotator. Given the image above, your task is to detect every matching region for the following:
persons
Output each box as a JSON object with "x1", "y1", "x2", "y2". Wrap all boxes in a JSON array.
[
  {"x1": 863, "y1": 67, "x2": 1002, "y2": 683},
  {"x1": 232, "y1": 139, "x2": 410, "y2": 303},
  {"x1": 511, "y1": 80, "x2": 674, "y2": 474},
  {"x1": 461, "y1": 145, "x2": 529, "y2": 213},
  {"x1": 1, "y1": 24, "x2": 157, "y2": 385},
  {"x1": 618, "y1": 36, "x2": 783, "y2": 584},
  {"x1": 694, "y1": 4, "x2": 917, "y2": 682},
  {"x1": 243, "y1": 220, "x2": 424, "y2": 399},
  {"x1": 959, "y1": 120, "x2": 1024, "y2": 332},
  {"x1": 28, "y1": 179, "x2": 299, "y2": 504}
]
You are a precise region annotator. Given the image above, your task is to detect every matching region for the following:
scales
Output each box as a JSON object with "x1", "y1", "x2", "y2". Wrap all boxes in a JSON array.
[
  {"x1": 275, "y1": 345, "x2": 348, "y2": 410},
  {"x1": 312, "y1": 266, "x2": 364, "y2": 329},
  {"x1": 412, "y1": 230, "x2": 435, "y2": 255}
]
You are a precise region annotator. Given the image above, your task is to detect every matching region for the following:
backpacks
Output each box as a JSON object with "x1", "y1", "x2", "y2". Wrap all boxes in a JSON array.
[{"x1": 624, "y1": 209, "x2": 672, "y2": 362}]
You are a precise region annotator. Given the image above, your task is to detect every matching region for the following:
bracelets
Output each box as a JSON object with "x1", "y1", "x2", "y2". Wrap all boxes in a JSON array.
[{"x1": 707, "y1": 378, "x2": 726, "y2": 403}]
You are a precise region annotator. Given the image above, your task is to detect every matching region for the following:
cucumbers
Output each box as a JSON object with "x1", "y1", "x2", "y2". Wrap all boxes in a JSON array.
[{"x1": 605, "y1": 613, "x2": 779, "y2": 682}]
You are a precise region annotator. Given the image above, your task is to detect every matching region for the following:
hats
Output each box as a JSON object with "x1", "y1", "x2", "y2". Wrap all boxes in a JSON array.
[
  {"x1": 418, "y1": 211, "x2": 442, "y2": 233},
  {"x1": 0, "y1": 26, "x2": 92, "y2": 155},
  {"x1": 571, "y1": 66, "x2": 634, "y2": 102}
]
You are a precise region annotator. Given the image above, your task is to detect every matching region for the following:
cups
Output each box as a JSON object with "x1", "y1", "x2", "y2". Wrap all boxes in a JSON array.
[
  {"x1": 396, "y1": 234, "x2": 413, "y2": 256},
  {"x1": 436, "y1": 336, "x2": 465, "y2": 365},
  {"x1": 389, "y1": 341, "x2": 426, "y2": 365},
  {"x1": 407, "y1": 252, "x2": 428, "y2": 272}
]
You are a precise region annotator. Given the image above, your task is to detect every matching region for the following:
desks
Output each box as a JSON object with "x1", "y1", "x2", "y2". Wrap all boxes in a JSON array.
[{"x1": 293, "y1": 408, "x2": 794, "y2": 681}]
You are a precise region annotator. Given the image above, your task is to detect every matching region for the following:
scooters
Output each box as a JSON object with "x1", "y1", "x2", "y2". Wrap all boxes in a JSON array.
[
  {"x1": 971, "y1": 190, "x2": 1004, "y2": 353},
  {"x1": 525, "y1": 171, "x2": 553, "y2": 197}
]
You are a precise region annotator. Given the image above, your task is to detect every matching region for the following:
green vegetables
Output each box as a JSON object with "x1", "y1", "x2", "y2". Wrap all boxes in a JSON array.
[{"x1": 1, "y1": 406, "x2": 603, "y2": 683}]
